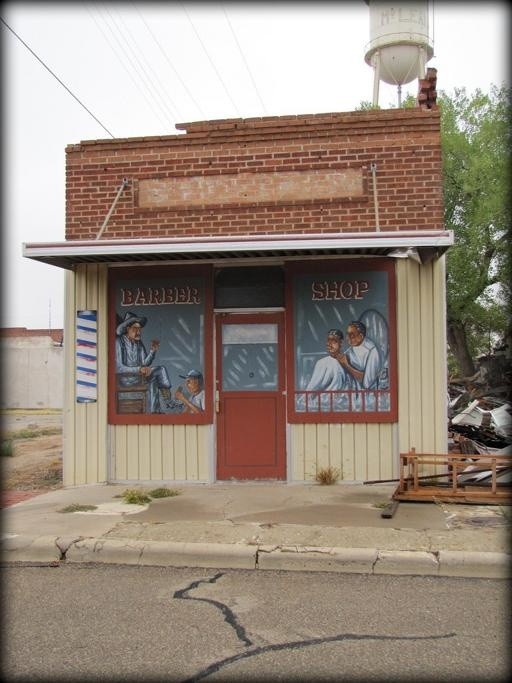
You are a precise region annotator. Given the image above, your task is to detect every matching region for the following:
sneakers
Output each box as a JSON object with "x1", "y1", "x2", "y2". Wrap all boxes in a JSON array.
[{"x1": 165, "y1": 399, "x2": 185, "y2": 409}]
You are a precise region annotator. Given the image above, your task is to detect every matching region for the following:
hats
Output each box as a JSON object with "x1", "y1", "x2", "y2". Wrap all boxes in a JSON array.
[
  {"x1": 327, "y1": 328, "x2": 343, "y2": 339},
  {"x1": 177, "y1": 369, "x2": 204, "y2": 382},
  {"x1": 115, "y1": 311, "x2": 147, "y2": 338}
]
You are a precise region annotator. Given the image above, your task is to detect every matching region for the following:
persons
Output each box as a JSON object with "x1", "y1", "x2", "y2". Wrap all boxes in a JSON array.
[
  {"x1": 173, "y1": 367, "x2": 206, "y2": 415},
  {"x1": 296, "y1": 328, "x2": 351, "y2": 411},
  {"x1": 115, "y1": 310, "x2": 183, "y2": 415},
  {"x1": 330, "y1": 320, "x2": 381, "y2": 410}
]
